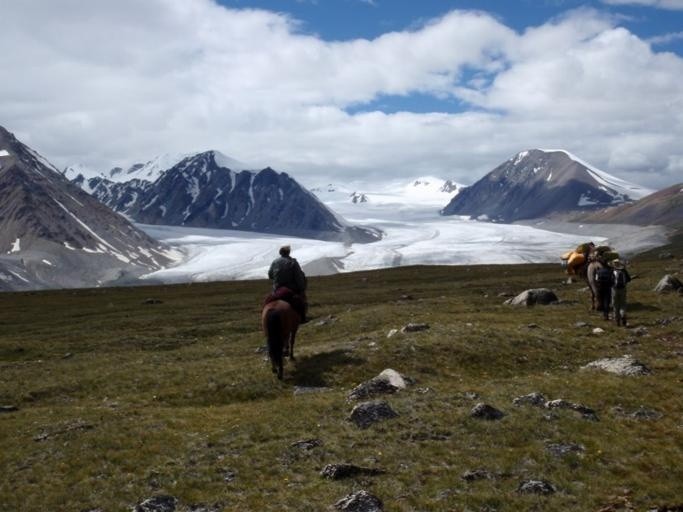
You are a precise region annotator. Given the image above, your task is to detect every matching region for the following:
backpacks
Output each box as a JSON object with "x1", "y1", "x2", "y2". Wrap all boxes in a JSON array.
[{"x1": 613, "y1": 270, "x2": 626, "y2": 289}]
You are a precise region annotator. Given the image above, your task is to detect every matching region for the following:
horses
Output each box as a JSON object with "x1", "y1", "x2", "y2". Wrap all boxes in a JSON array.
[
  {"x1": 581, "y1": 260, "x2": 603, "y2": 311},
  {"x1": 261, "y1": 277, "x2": 308, "y2": 380}
]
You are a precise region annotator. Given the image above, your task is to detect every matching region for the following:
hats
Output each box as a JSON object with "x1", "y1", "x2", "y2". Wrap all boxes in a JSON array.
[
  {"x1": 280, "y1": 244, "x2": 291, "y2": 251},
  {"x1": 608, "y1": 258, "x2": 624, "y2": 270}
]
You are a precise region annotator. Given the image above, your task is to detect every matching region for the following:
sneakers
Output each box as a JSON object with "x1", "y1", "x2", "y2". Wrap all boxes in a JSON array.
[{"x1": 617, "y1": 315, "x2": 627, "y2": 327}]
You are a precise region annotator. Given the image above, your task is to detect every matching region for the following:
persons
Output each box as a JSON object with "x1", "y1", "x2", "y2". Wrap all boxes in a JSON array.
[
  {"x1": 608, "y1": 259, "x2": 632, "y2": 325},
  {"x1": 266, "y1": 244, "x2": 305, "y2": 290}
]
